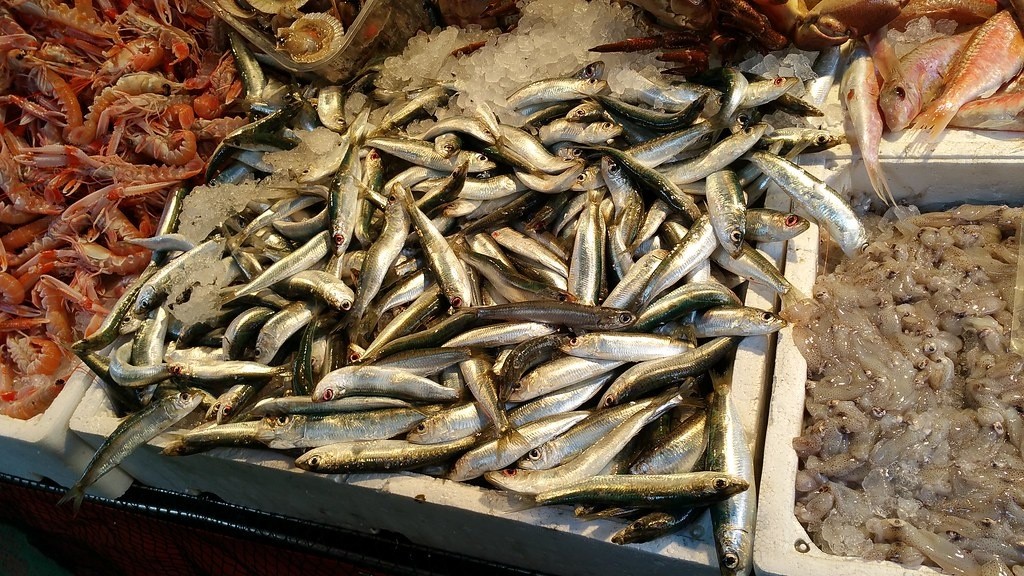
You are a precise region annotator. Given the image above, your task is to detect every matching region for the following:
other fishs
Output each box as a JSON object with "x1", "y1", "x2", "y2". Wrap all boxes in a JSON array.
[{"x1": 59, "y1": 0, "x2": 1024, "y2": 575}]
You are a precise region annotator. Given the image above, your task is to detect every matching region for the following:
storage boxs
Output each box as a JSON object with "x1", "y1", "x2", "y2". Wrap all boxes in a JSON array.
[{"x1": 0, "y1": 39, "x2": 1024, "y2": 576}]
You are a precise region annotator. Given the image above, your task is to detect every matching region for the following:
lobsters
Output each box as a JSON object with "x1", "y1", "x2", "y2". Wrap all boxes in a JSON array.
[{"x1": 0, "y1": 0, "x2": 249, "y2": 420}]
